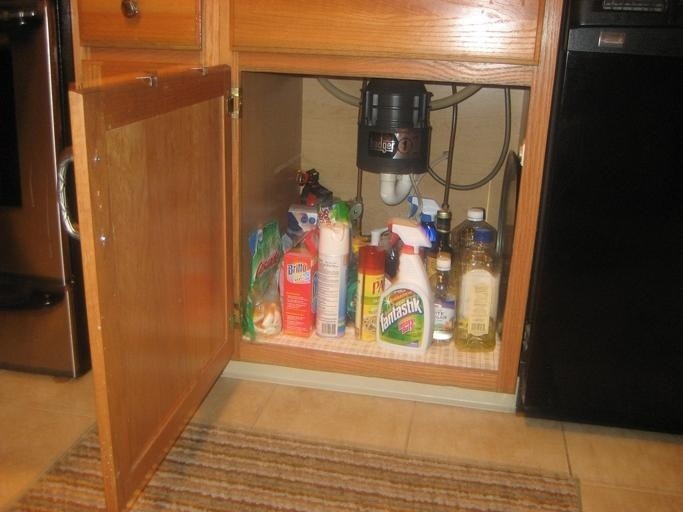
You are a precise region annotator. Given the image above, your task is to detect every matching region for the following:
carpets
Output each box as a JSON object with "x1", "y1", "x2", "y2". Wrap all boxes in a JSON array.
[{"x1": 2, "y1": 412, "x2": 585, "y2": 512}]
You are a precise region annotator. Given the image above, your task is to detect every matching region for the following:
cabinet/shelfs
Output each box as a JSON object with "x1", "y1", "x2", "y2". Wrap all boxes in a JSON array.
[{"x1": 66, "y1": 0, "x2": 554, "y2": 512}]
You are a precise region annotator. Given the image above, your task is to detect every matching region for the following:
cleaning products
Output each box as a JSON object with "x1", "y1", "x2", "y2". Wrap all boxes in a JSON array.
[
  {"x1": 406, "y1": 195, "x2": 442, "y2": 271},
  {"x1": 376, "y1": 218, "x2": 433, "y2": 352}
]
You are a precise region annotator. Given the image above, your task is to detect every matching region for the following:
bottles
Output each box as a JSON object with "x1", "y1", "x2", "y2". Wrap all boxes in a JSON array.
[{"x1": 425, "y1": 208, "x2": 497, "y2": 355}]
